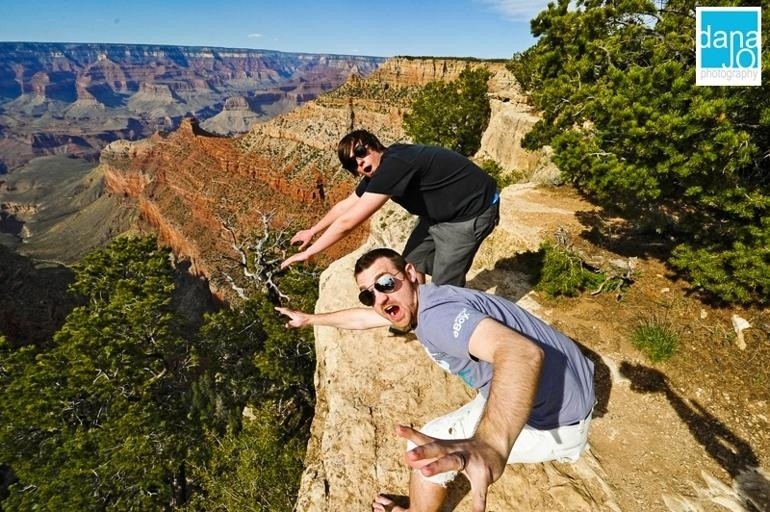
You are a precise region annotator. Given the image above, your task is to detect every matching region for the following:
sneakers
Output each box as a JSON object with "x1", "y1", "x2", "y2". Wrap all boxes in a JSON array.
[{"x1": 389, "y1": 322, "x2": 414, "y2": 334}]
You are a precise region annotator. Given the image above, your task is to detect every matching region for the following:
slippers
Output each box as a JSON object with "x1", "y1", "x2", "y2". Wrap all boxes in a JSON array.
[{"x1": 372, "y1": 494, "x2": 396, "y2": 512}]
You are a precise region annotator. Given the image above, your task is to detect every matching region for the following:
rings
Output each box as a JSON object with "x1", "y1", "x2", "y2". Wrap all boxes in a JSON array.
[{"x1": 453, "y1": 452, "x2": 466, "y2": 472}]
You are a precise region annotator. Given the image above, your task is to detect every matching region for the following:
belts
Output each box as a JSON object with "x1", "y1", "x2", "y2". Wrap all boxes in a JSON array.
[{"x1": 566, "y1": 408, "x2": 593, "y2": 426}]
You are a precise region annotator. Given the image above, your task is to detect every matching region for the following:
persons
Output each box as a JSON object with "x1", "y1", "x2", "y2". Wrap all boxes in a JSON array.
[
  {"x1": 274, "y1": 247, "x2": 599, "y2": 512},
  {"x1": 280, "y1": 130, "x2": 501, "y2": 336}
]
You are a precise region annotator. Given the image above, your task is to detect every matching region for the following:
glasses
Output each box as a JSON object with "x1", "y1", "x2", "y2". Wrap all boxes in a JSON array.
[
  {"x1": 349, "y1": 143, "x2": 369, "y2": 170},
  {"x1": 359, "y1": 271, "x2": 400, "y2": 305}
]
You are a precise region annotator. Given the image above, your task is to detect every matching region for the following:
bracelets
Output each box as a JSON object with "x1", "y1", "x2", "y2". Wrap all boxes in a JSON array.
[{"x1": 308, "y1": 226, "x2": 315, "y2": 243}]
